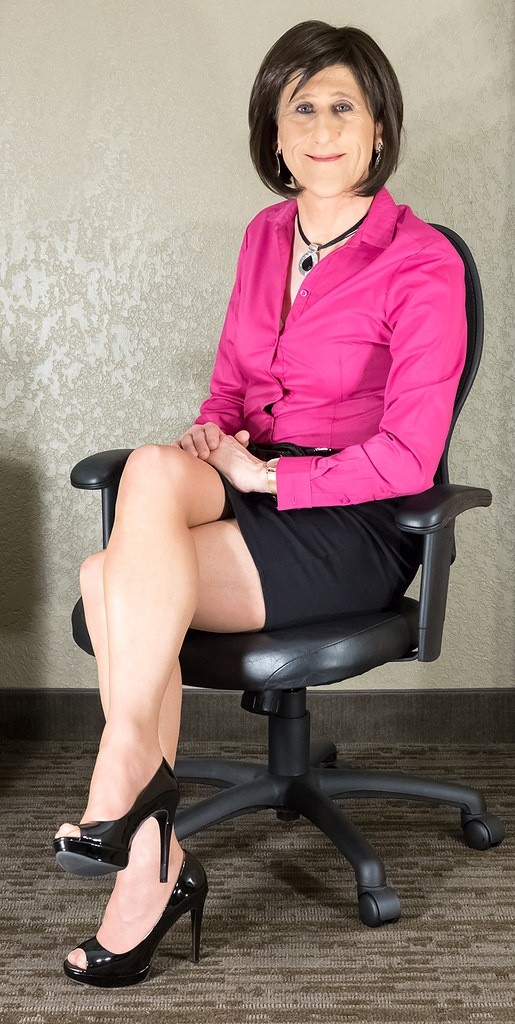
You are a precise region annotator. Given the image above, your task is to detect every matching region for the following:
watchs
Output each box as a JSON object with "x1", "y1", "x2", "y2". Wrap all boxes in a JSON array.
[{"x1": 265, "y1": 457, "x2": 282, "y2": 495}]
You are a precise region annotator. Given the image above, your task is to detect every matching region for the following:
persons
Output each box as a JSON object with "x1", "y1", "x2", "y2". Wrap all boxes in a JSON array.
[{"x1": 45, "y1": 16, "x2": 469, "y2": 989}]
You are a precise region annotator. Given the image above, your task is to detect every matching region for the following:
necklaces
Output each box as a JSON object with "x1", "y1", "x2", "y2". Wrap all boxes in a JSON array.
[{"x1": 292, "y1": 201, "x2": 369, "y2": 275}]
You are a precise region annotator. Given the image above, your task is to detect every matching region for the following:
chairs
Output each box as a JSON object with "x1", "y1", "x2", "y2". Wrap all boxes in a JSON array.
[{"x1": 69, "y1": 223, "x2": 508, "y2": 928}]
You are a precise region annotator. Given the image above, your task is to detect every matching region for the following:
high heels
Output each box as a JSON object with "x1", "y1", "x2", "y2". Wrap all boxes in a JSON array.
[
  {"x1": 64, "y1": 849, "x2": 208, "y2": 987},
  {"x1": 54, "y1": 757, "x2": 179, "y2": 882}
]
